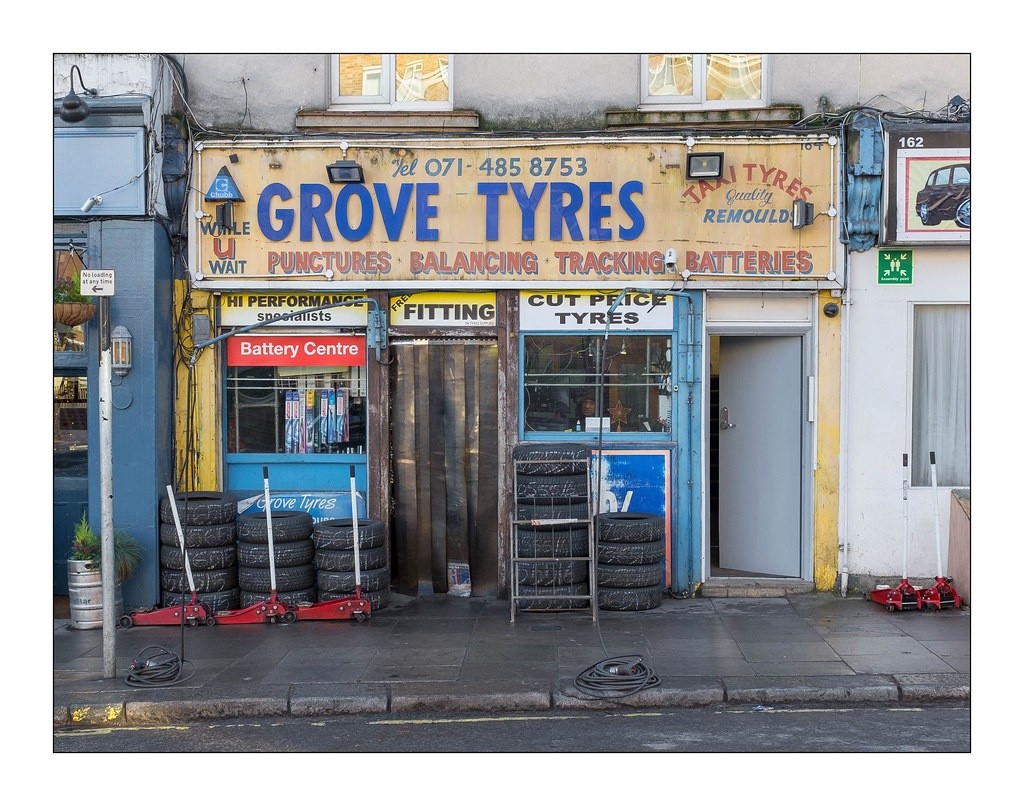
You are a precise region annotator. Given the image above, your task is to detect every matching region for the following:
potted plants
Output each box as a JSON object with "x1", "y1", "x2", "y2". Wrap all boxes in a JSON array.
[{"x1": 67, "y1": 510, "x2": 146, "y2": 630}]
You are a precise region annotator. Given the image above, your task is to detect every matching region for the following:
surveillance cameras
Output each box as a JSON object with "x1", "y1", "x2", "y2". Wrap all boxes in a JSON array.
[
  {"x1": 79, "y1": 196, "x2": 95, "y2": 212},
  {"x1": 664, "y1": 247, "x2": 677, "y2": 269}
]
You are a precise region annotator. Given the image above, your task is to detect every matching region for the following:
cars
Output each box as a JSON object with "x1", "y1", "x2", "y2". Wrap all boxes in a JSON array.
[{"x1": 915, "y1": 163, "x2": 970, "y2": 228}]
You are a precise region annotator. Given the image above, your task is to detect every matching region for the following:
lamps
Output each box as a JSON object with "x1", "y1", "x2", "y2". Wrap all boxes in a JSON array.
[
  {"x1": 216, "y1": 200, "x2": 234, "y2": 229},
  {"x1": 326, "y1": 160, "x2": 365, "y2": 183},
  {"x1": 792, "y1": 198, "x2": 814, "y2": 230},
  {"x1": 110, "y1": 325, "x2": 133, "y2": 377},
  {"x1": 60, "y1": 65, "x2": 98, "y2": 123},
  {"x1": 686, "y1": 152, "x2": 724, "y2": 180},
  {"x1": 81, "y1": 196, "x2": 103, "y2": 212}
]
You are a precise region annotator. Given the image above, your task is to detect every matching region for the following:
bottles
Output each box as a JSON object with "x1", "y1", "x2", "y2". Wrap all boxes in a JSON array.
[{"x1": 576, "y1": 419, "x2": 582, "y2": 432}]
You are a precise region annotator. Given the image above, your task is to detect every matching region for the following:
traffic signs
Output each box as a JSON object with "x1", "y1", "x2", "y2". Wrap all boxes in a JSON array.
[{"x1": 79, "y1": 268, "x2": 115, "y2": 297}]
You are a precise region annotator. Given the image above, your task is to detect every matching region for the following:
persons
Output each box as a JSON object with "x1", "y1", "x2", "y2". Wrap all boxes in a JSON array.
[{"x1": 576, "y1": 387, "x2": 598, "y2": 431}]
